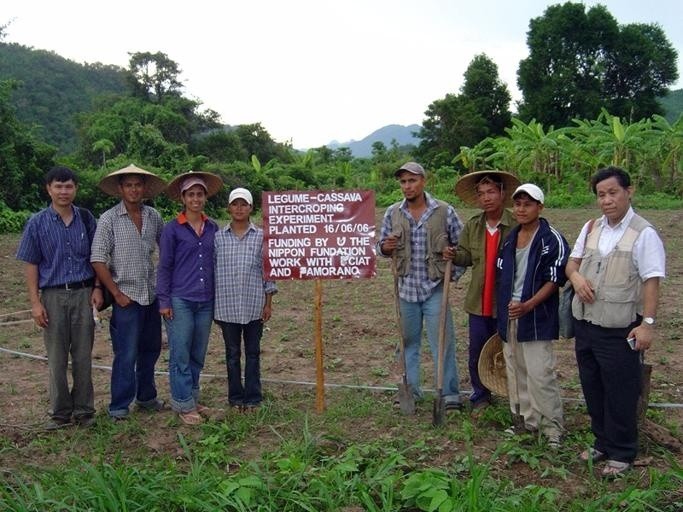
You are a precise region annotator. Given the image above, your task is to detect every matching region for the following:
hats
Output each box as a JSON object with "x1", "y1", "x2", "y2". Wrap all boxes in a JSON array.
[
  {"x1": 510, "y1": 183, "x2": 544, "y2": 205},
  {"x1": 455, "y1": 169, "x2": 522, "y2": 209},
  {"x1": 98, "y1": 163, "x2": 168, "y2": 199},
  {"x1": 166, "y1": 170, "x2": 223, "y2": 204},
  {"x1": 228, "y1": 187, "x2": 253, "y2": 206},
  {"x1": 477, "y1": 332, "x2": 509, "y2": 398},
  {"x1": 393, "y1": 161, "x2": 425, "y2": 178}
]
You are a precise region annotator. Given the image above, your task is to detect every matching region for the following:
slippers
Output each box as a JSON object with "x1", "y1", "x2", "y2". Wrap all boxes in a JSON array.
[{"x1": 179, "y1": 402, "x2": 211, "y2": 425}]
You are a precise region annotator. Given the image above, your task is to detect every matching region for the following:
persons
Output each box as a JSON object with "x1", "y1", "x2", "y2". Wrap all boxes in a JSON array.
[
  {"x1": 442, "y1": 165, "x2": 524, "y2": 405},
  {"x1": 89, "y1": 162, "x2": 173, "y2": 421},
  {"x1": 563, "y1": 164, "x2": 668, "y2": 479},
  {"x1": 210, "y1": 187, "x2": 280, "y2": 412},
  {"x1": 163, "y1": 168, "x2": 226, "y2": 423},
  {"x1": 495, "y1": 183, "x2": 575, "y2": 454},
  {"x1": 18, "y1": 166, "x2": 104, "y2": 432},
  {"x1": 374, "y1": 160, "x2": 467, "y2": 418}
]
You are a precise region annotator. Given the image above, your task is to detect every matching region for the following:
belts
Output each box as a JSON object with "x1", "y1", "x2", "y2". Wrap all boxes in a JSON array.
[{"x1": 49, "y1": 277, "x2": 95, "y2": 291}]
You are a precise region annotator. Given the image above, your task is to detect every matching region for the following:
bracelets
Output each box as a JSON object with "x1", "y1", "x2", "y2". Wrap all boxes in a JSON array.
[{"x1": 93, "y1": 284, "x2": 103, "y2": 290}]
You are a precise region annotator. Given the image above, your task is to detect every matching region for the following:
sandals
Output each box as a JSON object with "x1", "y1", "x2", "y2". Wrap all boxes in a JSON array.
[
  {"x1": 138, "y1": 400, "x2": 172, "y2": 413},
  {"x1": 601, "y1": 456, "x2": 634, "y2": 479},
  {"x1": 580, "y1": 445, "x2": 606, "y2": 462},
  {"x1": 112, "y1": 415, "x2": 131, "y2": 426}
]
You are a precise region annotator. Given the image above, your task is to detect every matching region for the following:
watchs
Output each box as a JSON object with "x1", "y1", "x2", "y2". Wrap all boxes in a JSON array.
[{"x1": 642, "y1": 317, "x2": 658, "y2": 326}]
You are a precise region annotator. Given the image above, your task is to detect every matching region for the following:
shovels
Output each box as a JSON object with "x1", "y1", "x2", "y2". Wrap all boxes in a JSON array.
[
  {"x1": 392, "y1": 234, "x2": 417, "y2": 416},
  {"x1": 510, "y1": 318, "x2": 526, "y2": 434},
  {"x1": 432, "y1": 258, "x2": 454, "y2": 428}
]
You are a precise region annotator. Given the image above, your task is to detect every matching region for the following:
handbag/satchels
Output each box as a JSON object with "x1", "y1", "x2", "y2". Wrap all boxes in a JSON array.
[
  {"x1": 558, "y1": 218, "x2": 596, "y2": 340},
  {"x1": 77, "y1": 207, "x2": 114, "y2": 312}
]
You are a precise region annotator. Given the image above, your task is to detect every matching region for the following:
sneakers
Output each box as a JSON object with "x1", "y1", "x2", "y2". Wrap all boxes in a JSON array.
[
  {"x1": 76, "y1": 416, "x2": 94, "y2": 433},
  {"x1": 391, "y1": 400, "x2": 400, "y2": 410},
  {"x1": 444, "y1": 400, "x2": 462, "y2": 417},
  {"x1": 503, "y1": 425, "x2": 533, "y2": 440},
  {"x1": 231, "y1": 404, "x2": 257, "y2": 416},
  {"x1": 44, "y1": 417, "x2": 74, "y2": 429},
  {"x1": 547, "y1": 435, "x2": 563, "y2": 448},
  {"x1": 470, "y1": 399, "x2": 490, "y2": 421}
]
